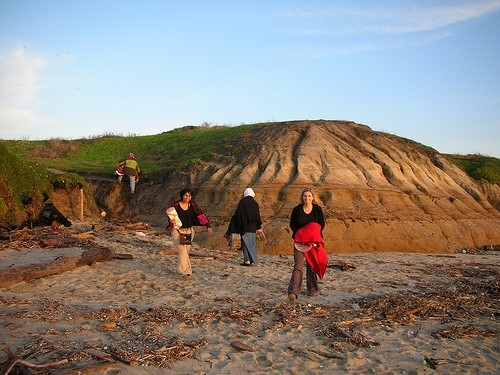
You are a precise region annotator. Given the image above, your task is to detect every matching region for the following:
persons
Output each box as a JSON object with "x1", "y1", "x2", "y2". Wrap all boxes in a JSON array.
[
  {"x1": 116, "y1": 153, "x2": 141, "y2": 195},
  {"x1": 167, "y1": 188, "x2": 214, "y2": 277},
  {"x1": 288, "y1": 190, "x2": 328, "y2": 301},
  {"x1": 224, "y1": 187, "x2": 263, "y2": 266}
]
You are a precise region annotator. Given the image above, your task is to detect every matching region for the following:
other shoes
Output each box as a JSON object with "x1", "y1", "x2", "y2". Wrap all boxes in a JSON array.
[
  {"x1": 309, "y1": 291, "x2": 317, "y2": 296},
  {"x1": 130, "y1": 190, "x2": 135, "y2": 193},
  {"x1": 240, "y1": 262, "x2": 250, "y2": 267},
  {"x1": 288, "y1": 294, "x2": 296, "y2": 302},
  {"x1": 183, "y1": 275, "x2": 188, "y2": 280},
  {"x1": 116, "y1": 180, "x2": 121, "y2": 183}
]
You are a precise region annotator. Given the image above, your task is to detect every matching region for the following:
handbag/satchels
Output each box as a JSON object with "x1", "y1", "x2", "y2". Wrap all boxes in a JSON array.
[{"x1": 179, "y1": 233, "x2": 192, "y2": 245}]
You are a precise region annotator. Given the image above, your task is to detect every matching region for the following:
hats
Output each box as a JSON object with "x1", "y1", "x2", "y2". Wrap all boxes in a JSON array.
[{"x1": 127, "y1": 153, "x2": 135, "y2": 158}]
